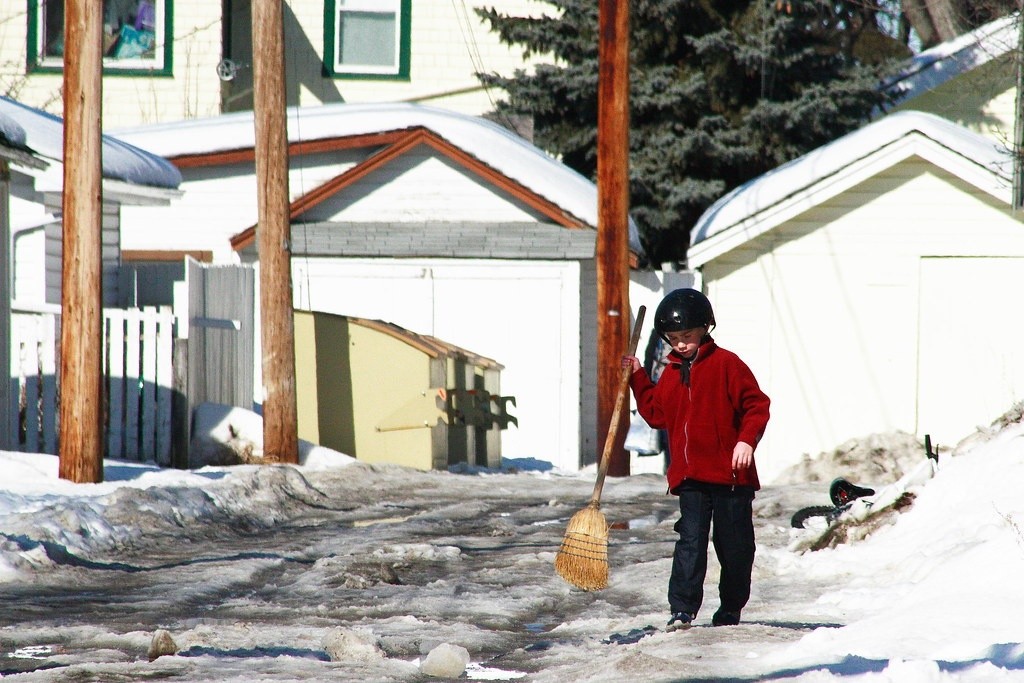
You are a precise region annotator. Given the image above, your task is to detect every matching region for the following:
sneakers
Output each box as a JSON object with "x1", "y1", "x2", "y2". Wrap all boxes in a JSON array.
[
  {"x1": 666, "y1": 612, "x2": 691, "y2": 633},
  {"x1": 712, "y1": 614, "x2": 739, "y2": 626}
]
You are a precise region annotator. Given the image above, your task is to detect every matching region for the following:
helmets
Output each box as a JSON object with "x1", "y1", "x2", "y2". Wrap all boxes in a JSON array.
[{"x1": 654, "y1": 288, "x2": 715, "y2": 331}]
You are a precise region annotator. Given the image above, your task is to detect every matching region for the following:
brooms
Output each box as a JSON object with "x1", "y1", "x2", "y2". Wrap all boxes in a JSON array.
[{"x1": 551, "y1": 306, "x2": 646, "y2": 592}]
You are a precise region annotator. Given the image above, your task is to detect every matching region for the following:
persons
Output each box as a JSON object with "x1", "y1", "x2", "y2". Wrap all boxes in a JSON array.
[{"x1": 620, "y1": 288, "x2": 770, "y2": 632}]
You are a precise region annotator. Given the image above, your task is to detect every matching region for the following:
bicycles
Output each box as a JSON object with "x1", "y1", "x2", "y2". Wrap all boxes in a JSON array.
[{"x1": 790, "y1": 434, "x2": 938, "y2": 526}]
